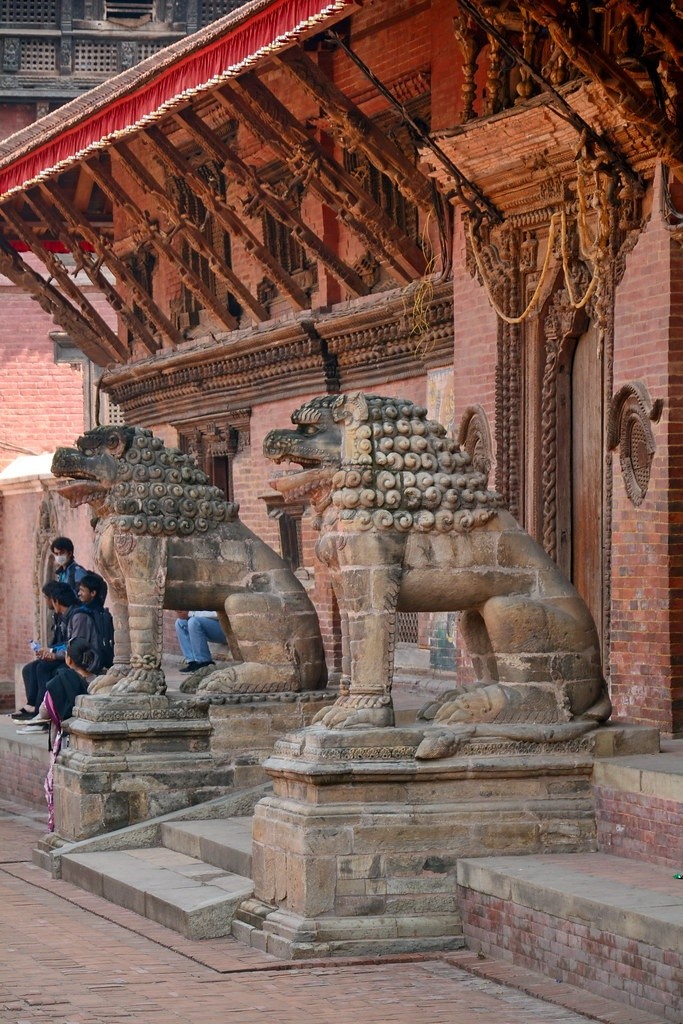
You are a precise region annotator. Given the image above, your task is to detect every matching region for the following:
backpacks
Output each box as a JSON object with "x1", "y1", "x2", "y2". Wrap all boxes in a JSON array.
[{"x1": 73, "y1": 609, "x2": 113, "y2": 667}]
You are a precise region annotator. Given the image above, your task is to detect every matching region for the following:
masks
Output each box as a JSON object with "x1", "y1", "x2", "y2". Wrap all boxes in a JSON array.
[{"x1": 54, "y1": 554, "x2": 69, "y2": 565}]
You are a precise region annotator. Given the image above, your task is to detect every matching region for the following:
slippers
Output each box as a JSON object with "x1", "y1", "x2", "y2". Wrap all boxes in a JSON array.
[{"x1": 11, "y1": 708, "x2": 32, "y2": 720}]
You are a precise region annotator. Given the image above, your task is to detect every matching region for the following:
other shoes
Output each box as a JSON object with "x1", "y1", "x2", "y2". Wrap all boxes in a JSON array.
[
  {"x1": 24, "y1": 713, "x2": 52, "y2": 724},
  {"x1": 17, "y1": 725, "x2": 47, "y2": 733},
  {"x1": 179, "y1": 661, "x2": 216, "y2": 674}
]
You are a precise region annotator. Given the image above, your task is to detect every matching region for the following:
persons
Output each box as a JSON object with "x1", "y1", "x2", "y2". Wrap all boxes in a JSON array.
[
  {"x1": 16, "y1": 581, "x2": 104, "y2": 735},
  {"x1": 50, "y1": 536, "x2": 90, "y2": 601},
  {"x1": 174, "y1": 608, "x2": 228, "y2": 675},
  {"x1": 38, "y1": 636, "x2": 101, "y2": 832},
  {"x1": 75, "y1": 575, "x2": 116, "y2": 675},
  {"x1": 11, "y1": 581, "x2": 66, "y2": 720}
]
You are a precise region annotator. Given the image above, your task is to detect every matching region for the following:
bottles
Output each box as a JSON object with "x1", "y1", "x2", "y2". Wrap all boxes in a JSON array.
[{"x1": 27, "y1": 639, "x2": 43, "y2": 655}]
